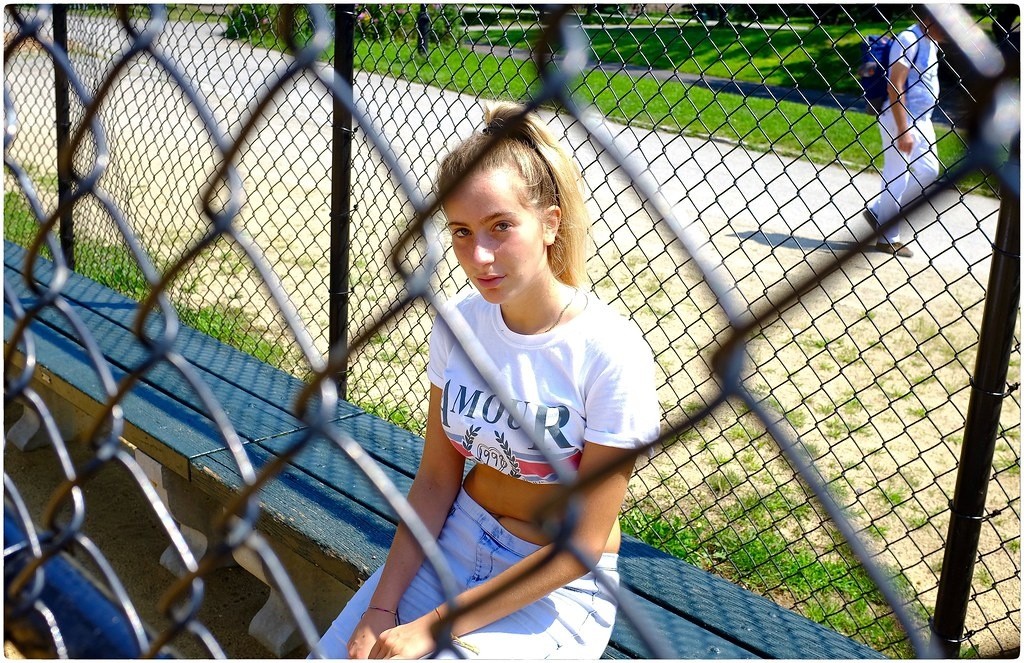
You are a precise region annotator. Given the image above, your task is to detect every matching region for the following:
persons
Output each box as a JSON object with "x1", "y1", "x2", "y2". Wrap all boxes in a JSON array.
[
  {"x1": 303, "y1": 106, "x2": 662, "y2": 660},
  {"x1": 862, "y1": 13, "x2": 948, "y2": 259}
]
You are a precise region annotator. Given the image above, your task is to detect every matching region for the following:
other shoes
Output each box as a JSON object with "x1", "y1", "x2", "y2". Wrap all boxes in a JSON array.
[
  {"x1": 875, "y1": 243, "x2": 913, "y2": 258},
  {"x1": 863, "y1": 208, "x2": 878, "y2": 231}
]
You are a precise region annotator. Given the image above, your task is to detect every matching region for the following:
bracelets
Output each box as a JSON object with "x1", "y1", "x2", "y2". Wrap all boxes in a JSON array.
[
  {"x1": 368, "y1": 606, "x2": 396, "y2": 616},
  {"x1": 435, "y1": 607, "x2": 480, "y2": 655}
]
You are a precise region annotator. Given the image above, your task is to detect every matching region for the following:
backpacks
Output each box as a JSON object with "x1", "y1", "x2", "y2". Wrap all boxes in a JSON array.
[{"x1": 857, "y1": 29, "x2": 920, "y2": 115}]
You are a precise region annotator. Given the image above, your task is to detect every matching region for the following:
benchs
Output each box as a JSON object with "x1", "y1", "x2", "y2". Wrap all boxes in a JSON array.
[{"x1": 4, "y1": 243, "x2": 887, "y2": 660}]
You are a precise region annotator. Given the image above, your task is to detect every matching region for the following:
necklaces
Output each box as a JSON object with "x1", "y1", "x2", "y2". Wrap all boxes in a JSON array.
[{"x1": 543, "y1": 287, "x2": 578, "y2": 333}]
